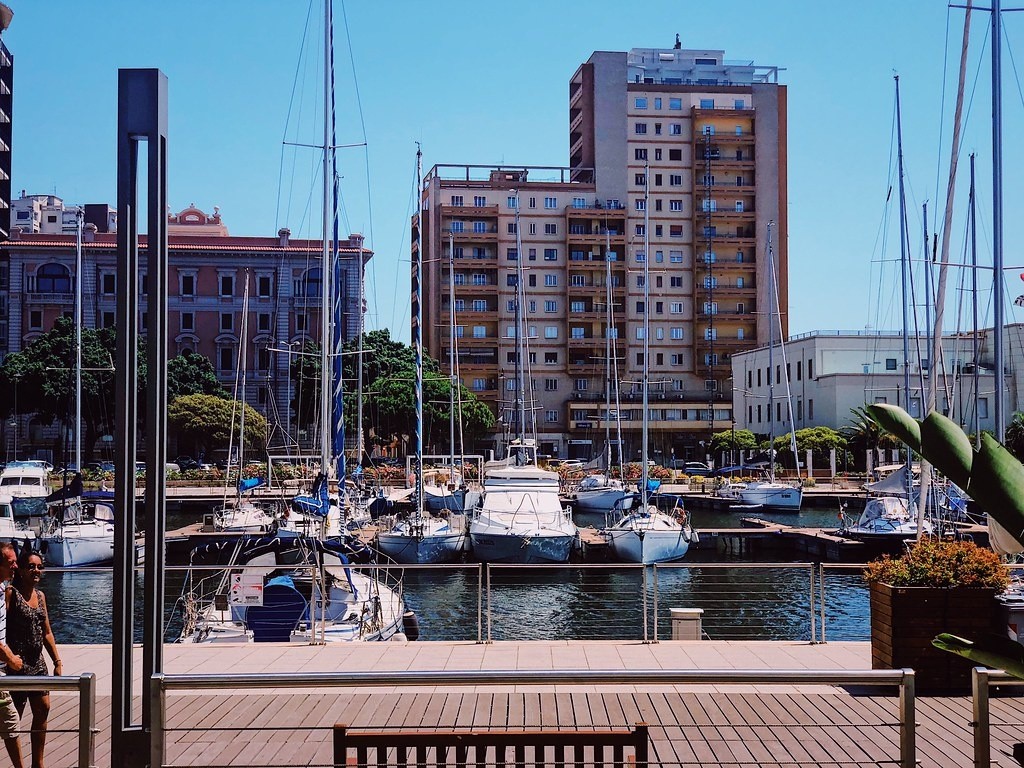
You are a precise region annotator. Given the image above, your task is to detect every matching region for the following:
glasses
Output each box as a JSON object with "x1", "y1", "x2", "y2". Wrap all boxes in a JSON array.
[{"x1": 19, "y1": 564, "x2": 45, "y2": 570}]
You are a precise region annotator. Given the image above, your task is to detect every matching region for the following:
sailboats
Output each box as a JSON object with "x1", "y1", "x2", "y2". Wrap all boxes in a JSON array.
[{"x1": 0, "y1": 0, "x2": 1022, "y2": 642}]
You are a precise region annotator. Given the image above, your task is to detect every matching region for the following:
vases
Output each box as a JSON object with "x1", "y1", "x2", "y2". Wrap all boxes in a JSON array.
[{"x1": 869, "y1": 579, "x2": 997, "y2": 697}]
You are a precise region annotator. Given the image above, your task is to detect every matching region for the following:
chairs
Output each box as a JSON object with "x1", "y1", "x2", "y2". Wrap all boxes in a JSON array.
[{"x1": 245, "y1": 585, "x2": 309, "y2": 642}]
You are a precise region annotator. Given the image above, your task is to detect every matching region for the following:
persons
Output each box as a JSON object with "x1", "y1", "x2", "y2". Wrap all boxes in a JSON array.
[
  {"x1": 6, "y1": 549, "x2": 61, "y2": 768},
  {"x1": 0, "y1": 543, "x2": 25, "y2": 768}
]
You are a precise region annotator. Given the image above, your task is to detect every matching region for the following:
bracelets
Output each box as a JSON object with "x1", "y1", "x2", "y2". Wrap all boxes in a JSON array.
[{"x1": 54, "y1": 660, "x2": 61, "y2": 664}]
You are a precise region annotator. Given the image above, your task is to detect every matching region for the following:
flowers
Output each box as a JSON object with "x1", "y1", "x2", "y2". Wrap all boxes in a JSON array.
[{"x1": 852, "y1": 535, "x2": 1014, "y2": 596}]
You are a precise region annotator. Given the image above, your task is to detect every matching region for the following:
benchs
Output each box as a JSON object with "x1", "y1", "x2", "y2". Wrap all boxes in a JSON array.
[{"x1": 332, "y1": 721, "x2": 649, "y2": 768}]
